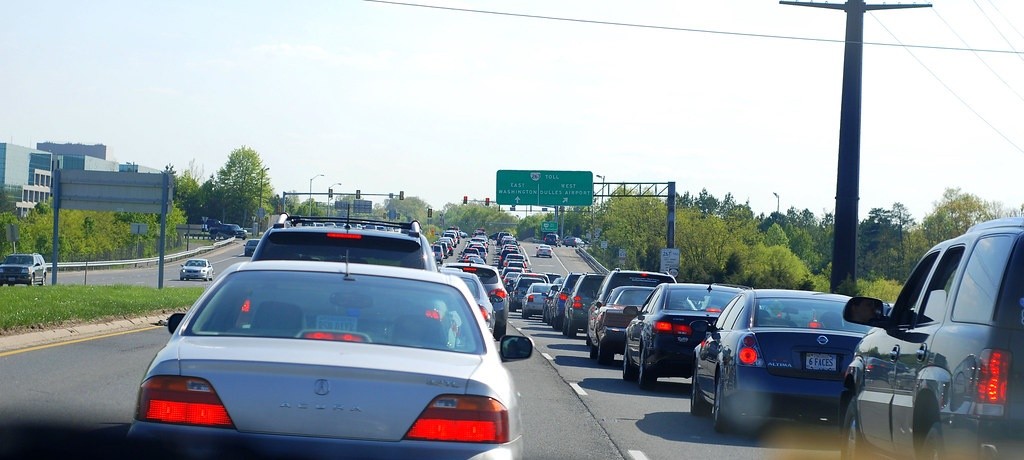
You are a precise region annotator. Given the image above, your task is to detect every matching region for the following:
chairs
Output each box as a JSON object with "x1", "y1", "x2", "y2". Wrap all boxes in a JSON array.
[
  {"x1": 249, "y1": 300, "x2": 446, "y2": 352},
  {"x1": 758, "y1": 309, "x2": 774, "y2": 325}
]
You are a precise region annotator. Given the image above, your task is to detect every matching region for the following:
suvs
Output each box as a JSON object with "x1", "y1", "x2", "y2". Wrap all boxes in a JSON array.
[
  {"x1": 440, "y1": 261, "x2": 514, "y2": 341},
  {"x1": 551, "y1": 272, "x2": 607, "y2": 338},
  {"x1": 841, "y1": 217, "x2": 1024, "y2": 460},
  {"x1": 0, "y1": 252, "x2": 47, "y2": 286},
  {"x1": 587, "y1": 264, "x2": 678, "y2": 359},
  {"x1": 250, "y1": 212, "x2": 437, "y2": 274}
]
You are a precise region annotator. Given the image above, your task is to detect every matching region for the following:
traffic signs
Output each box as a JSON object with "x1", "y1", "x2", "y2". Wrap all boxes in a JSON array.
[{"x1": 496, "y1": 170, "x2": 593, "y2": 206}]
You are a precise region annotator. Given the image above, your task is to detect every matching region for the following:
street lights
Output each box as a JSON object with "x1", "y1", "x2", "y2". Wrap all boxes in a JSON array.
[
  {"x1": 596, "y1": 175, "x2": 605, "y2": 214},
  {"x1": 310, "y1": 174, "x2": 341, "y2": 215}
]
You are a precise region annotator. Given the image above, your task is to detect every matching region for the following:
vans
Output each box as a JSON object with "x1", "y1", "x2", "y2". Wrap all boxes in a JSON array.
[{"x1": 243, "y1": 239, "x2": 260, "y2": 256}]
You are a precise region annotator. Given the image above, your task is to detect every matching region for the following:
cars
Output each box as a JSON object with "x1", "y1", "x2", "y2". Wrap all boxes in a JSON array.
[
  {"x1": 126, "y1": 260, "x2": 534, "y2": 460},
  {"x1": 495, "y1": 231, "x2": 584, "y2": 320},
  {"x1": 432, "y1": 225, "x2": 490, "y2": 266},
  {"x1": 595, "y1": 282, "x2": 745, "y2": 389},
  {"x1": 203, "y1": 219, "x2": 220, "y2": 231},
  {"x1": 688, "y1": 288, "x2": 874, "y2": 435},
  {"x1": 180, "y1": 260, "x2": 214, "y2": 281}
]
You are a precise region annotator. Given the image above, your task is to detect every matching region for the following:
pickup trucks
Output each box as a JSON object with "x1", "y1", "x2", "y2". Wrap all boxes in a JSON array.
[{"x1": 209, "y1": 224, "x2": 247, "y2": 240}]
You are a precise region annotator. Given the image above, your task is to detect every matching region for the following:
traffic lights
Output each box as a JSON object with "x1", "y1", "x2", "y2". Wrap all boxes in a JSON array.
[
  {"x1": 486, "y1": 198, "x2": 489, "y2": 205},
  {"x1": 464, "y1": 196, "x2": 467, "y2": 204}
]
